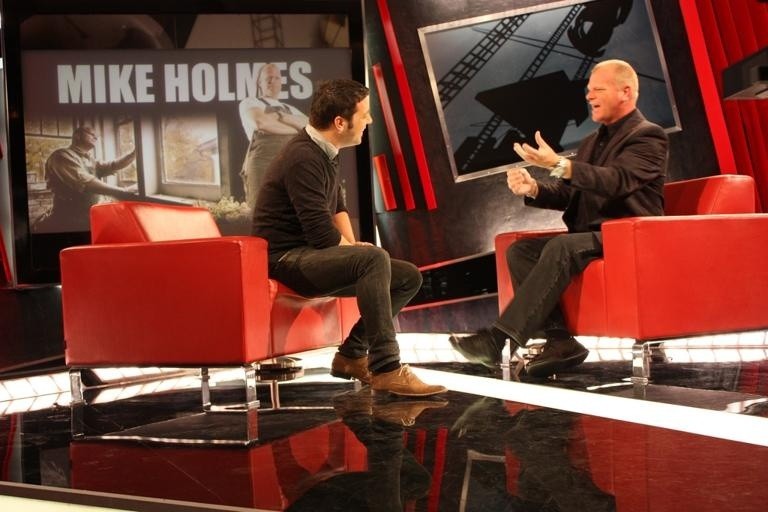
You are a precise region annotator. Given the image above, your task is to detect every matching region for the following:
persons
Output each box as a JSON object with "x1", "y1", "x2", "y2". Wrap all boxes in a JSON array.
[
  {"x1": 251, "y1": 80, "x2": 448, "y2": 399},
  {"x1": 449, "y1": 397, "x2": 618, "y2": 512},
  {"x1": 449, "y1": 57, "x2": 671, "y2": 373},
  {"x1": 287, "y1": 383, "x2": 433, "y2": 512},
  {"x1": 239, "y1": 62, "x2": 308, "y2": 207},
  {"x1": 45, "y1": 126, "x2": 138, "y2": 230}
]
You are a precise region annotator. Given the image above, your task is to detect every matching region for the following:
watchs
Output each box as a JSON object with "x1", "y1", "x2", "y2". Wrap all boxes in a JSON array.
[{"x1": 550, "y1": 155, "x2": 569, "y2": 179}]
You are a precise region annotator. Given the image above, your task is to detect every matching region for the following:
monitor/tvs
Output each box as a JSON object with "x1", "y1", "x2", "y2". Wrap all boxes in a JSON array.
[{"x1": 0, "y1": 0, "x2": 375, "y2": 286}]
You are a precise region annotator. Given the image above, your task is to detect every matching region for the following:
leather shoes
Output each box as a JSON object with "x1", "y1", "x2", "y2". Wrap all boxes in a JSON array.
[
  {"x1": 330, "y1": 352, "x2": 372, "y2": 384},
  {"x1": 371, "y1": 362, "x2": 449, "y2": 396}
]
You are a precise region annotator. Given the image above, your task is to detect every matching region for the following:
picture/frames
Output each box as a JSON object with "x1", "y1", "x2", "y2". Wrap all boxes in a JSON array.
[{"x1": 417, "y1": 1, "x2": 683, "y2": 184}]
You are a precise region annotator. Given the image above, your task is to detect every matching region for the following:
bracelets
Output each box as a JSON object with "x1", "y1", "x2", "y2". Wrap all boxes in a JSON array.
[{"x1": 274, "y1": 107, "x2": 283, "y2": 119}]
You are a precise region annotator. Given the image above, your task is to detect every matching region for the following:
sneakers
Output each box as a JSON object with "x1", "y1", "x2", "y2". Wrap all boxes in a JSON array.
[
  {"x1": 450, "y1": 328, "x2": 502, "y2": 368},
  {"x1": 526, "y1": 334, "x2": 588, "y2": 377}
]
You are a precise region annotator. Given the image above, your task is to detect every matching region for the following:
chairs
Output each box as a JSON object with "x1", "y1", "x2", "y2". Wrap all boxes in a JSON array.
[
  {"x1": 495, "y1": 174, "x2": 768, "y2": 386},
  {"x1": 60, "y1": 199, "x2": 363, "y2": 449}
]
